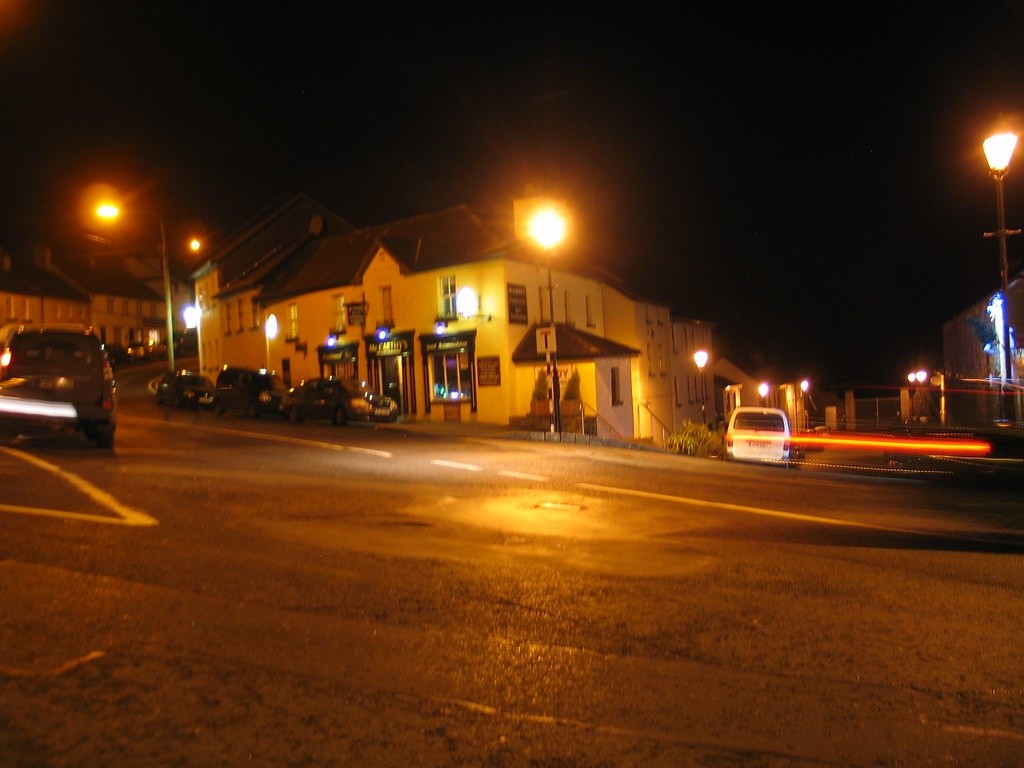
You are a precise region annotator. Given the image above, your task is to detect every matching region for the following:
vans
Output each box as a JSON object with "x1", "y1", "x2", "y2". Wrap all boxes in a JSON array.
[{"x1": 724, "y1": 406, "x2": 789, "y2": 467}]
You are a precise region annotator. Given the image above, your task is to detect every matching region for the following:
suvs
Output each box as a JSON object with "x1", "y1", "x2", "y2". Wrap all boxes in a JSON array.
[
  {"x1": 0, "y1": 323, "x2": 117, "y2": 449},
  {"x1": 216, "y1": 364, "x2": 288, "y2": 422}
]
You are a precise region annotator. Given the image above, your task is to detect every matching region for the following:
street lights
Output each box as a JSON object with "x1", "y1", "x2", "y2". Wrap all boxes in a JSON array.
[
  {"x1": 982, "y1": 129, "x2": 1020, "y2": 425},
  {"x1": 800, "y1": 380, "x2": 809, "y2": 430},
  {"x1": 526, "y1": 206, "x2": 562, "y2": 430},
  {"x1": 694, "y1": 350, "x2": 708, "y2": 426},
  {"x1": 914, "y1": 372, "x2": 927, "y2": 420},
  {"x1": 95, "y1": 202, "x2": 175, "y2": 372}
]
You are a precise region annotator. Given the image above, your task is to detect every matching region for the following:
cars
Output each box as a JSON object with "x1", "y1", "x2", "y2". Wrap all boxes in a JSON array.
[
  {"x1": 157, "y1": 369, "x2": 219, "y2": 412},
  {"x1": 105, "y1": 328, "x2": 198, "y2": 372},
  {"x1": 286, "y1": 377, "x2": 398, "y2": 426}
]
